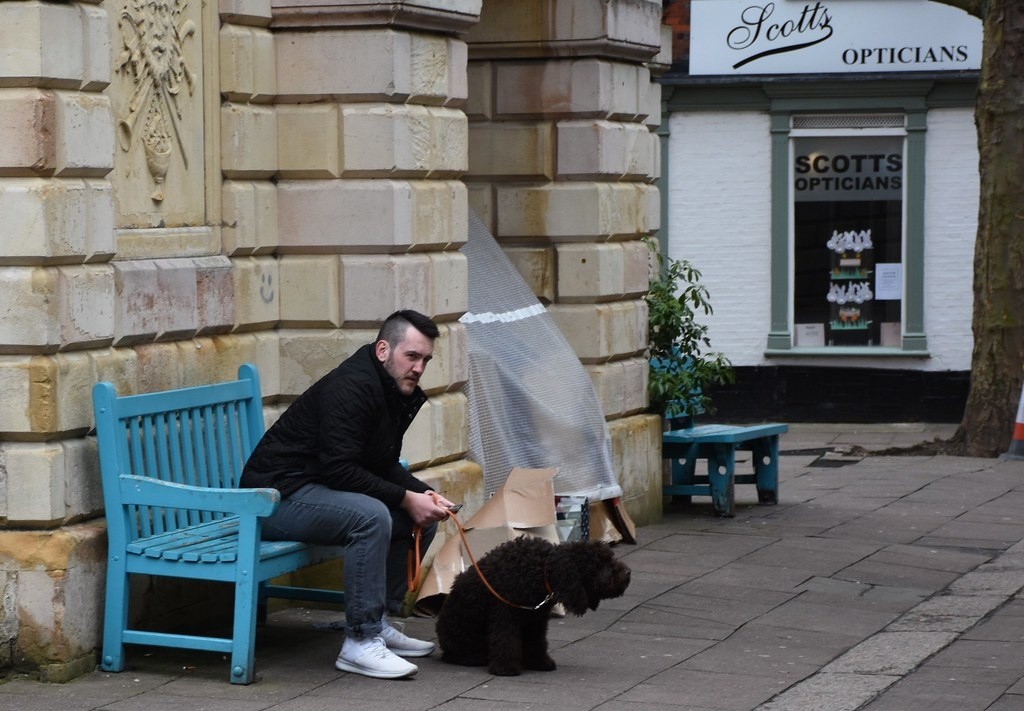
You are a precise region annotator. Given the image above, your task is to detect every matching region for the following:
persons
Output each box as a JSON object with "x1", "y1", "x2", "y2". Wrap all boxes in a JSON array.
[{"x1": 237, "y1": 309, "x2": 456, "y2": 680}]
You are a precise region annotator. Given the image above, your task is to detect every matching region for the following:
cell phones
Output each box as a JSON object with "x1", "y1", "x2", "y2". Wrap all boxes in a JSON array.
[{"x1": 448, "y1": 505, "x2": 463, "y2": 511}]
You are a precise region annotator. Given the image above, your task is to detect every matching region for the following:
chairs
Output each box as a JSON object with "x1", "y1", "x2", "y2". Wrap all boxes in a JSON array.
[
  {"x1": 92, "y1": 361, "x2": 410, "y2": 684},
  {"x1": 647, "y1": 344, "x2": 790, "y2": 518}
]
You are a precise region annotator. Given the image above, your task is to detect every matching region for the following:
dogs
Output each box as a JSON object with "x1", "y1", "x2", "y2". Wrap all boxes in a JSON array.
[{"x1": 434, "y1": 534, "x2": 631, "y2": 677}]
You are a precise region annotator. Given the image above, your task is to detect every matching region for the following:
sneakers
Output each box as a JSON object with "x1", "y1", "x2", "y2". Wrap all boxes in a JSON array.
[
  {"x1": 334, "y1": 637, "x2": 419, "y2": 679},
  {"x1": 375, "y1": 618, "x2": 434, "y2": 657}
]
